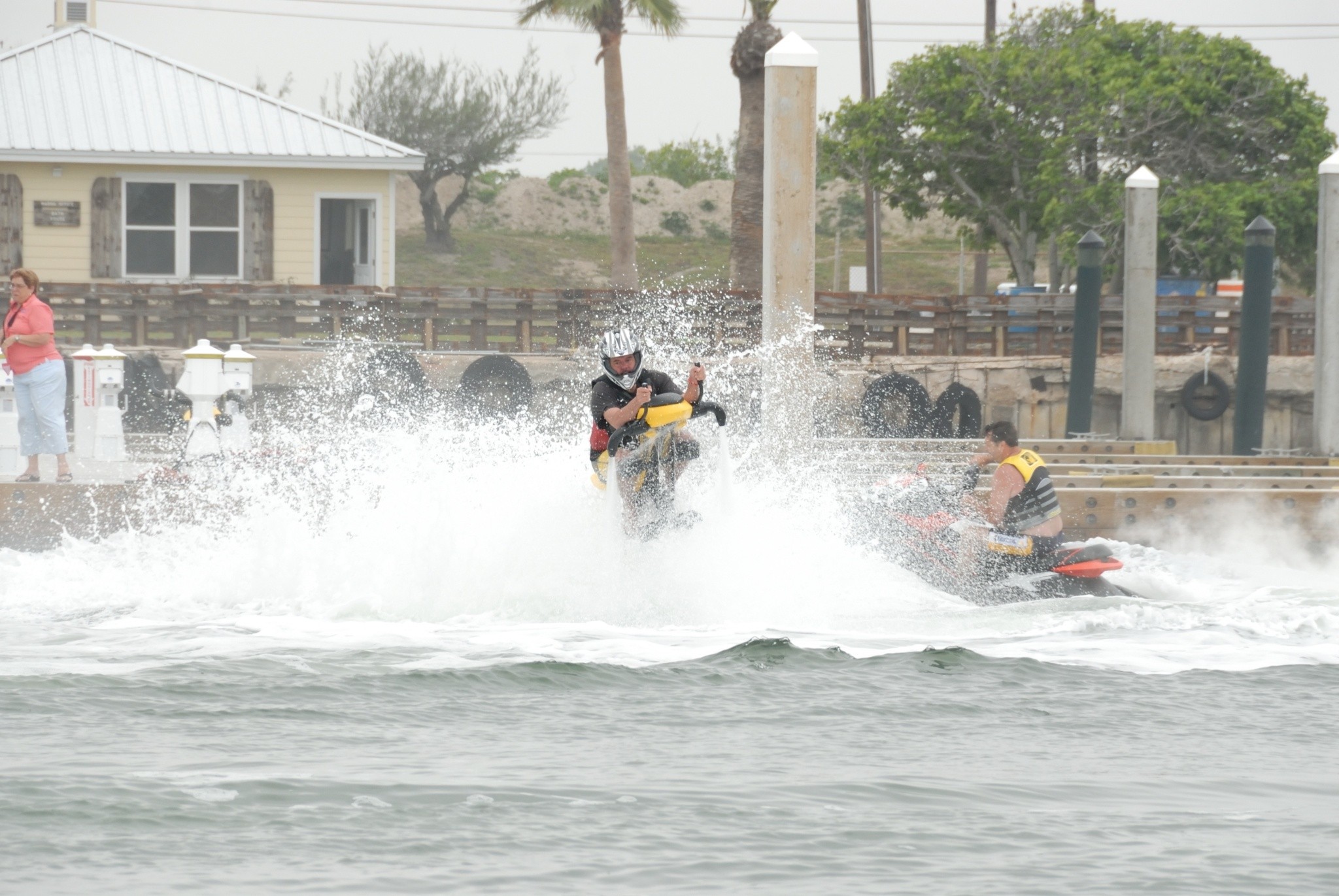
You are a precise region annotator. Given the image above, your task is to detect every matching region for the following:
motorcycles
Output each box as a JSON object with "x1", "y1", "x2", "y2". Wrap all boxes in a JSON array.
[{"x1": 842, "y1": 457, "x2": 1127, "y2": 604}]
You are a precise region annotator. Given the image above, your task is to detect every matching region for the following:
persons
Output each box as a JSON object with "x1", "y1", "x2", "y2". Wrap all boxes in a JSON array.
[
  {"x1": 589, "y1": 328, "x2": 706, "y2": 533},
  {"x1": 957, "y1": 421, "x2": 1063, "y2": 574},
  {"x1": 0, "y1": 268, "x2": 74, "y2": 482}
]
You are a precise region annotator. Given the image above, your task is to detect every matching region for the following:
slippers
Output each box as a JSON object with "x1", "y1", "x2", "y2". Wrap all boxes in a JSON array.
[
  {"x1": 56, "y1": 474, "x2": 73, "y2": 483},
  {"x1": 15, "y1": 474, "x2": 40, "y2": 483}
]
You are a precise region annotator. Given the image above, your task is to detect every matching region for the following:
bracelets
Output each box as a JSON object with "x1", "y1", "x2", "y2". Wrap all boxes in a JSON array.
[
  {"x1": 688, "y1": 378, "x2": 698, "y2": 386},
  {"x1": 15, "y1": 335, "x2": 19, "y2": 342}
]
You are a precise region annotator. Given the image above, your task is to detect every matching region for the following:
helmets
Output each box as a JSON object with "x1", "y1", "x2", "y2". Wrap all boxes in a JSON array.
[{"x1": 597, "y1": 329, "x2": 645, "y2": 391}]
